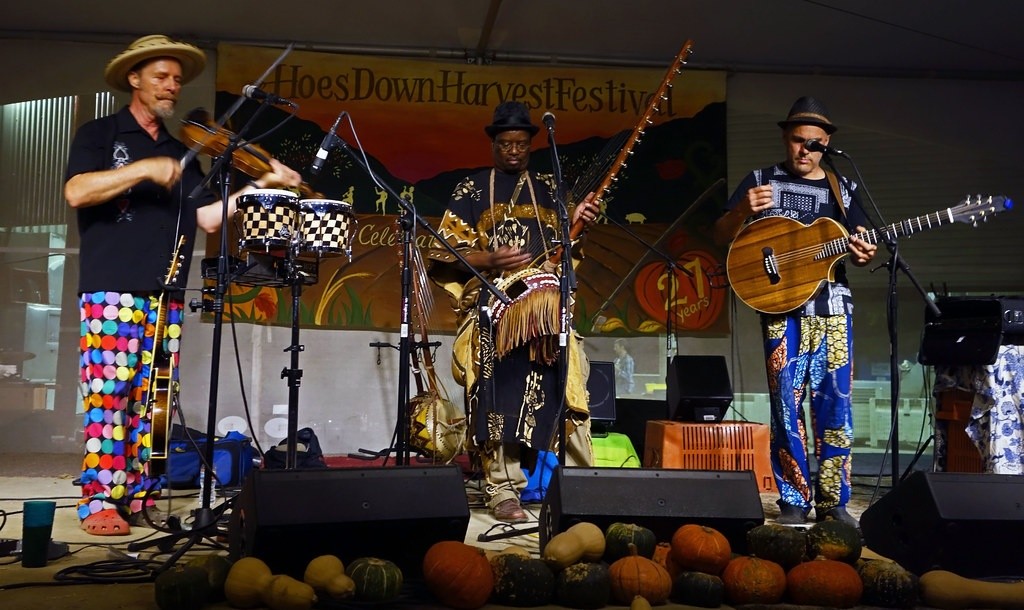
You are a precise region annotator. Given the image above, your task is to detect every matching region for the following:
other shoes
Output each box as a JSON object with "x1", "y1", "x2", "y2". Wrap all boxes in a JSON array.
[
  {"x1": 775, "y1": 499, "x2": 811, "y2": 523},
  {"x1": 815, "y1": 506, "x2": 860, "y2": 528}
]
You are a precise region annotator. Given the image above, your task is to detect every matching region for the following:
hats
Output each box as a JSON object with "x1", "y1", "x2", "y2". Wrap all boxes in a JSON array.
[
  {"x1": 104, "y1": 35, "x2": 208, "y2": 92},
  {"x1": 485, "y1": 102, "x2": 540, "y2": 139},
  {"x1": 777, "y1": 96, "x2": 838, "y2": 135}
]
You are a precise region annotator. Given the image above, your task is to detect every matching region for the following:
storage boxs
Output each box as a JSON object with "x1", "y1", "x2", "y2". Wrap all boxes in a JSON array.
[
  {"x1": 937, "y1": 391, "x2": 985, "y2": 473},
  {"x1": 593, "y1": 432, "x2": 641, "y2": 466},
  {"x1": 643, "y1": 419, "x2": 779, "y2": 493}
]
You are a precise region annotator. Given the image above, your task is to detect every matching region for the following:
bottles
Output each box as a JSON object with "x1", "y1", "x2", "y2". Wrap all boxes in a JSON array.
[{"x1": 199, "y1": 463, "x2": 216, "y2": 507}]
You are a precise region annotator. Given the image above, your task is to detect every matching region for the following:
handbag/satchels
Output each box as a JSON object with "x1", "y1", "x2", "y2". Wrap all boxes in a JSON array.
[{"x1": 167, "y1": 422, "x2": 254, "y2": 488}]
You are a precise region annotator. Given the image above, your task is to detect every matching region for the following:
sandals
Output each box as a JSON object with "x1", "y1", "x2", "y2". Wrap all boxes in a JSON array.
[
  {"x1": 121, "y1": 507, "x2": 181, "y2": 528},
  {"x1": 81, "y1": 508, "x2": 130, "y2": 534}
]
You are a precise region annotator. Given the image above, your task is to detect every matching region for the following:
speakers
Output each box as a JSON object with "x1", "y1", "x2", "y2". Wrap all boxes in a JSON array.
[
  {"x1": 667, "y1": 356, "x2": 734, "y2": 425},
  {"x1": 859, "y1": 470, "x2": 1024, "y2": 581},
  {"x1": 538, "y1": 465, "x2": 766, "y2": 559},
  {"x1": 226, "y1": 470, "x2": 469, "y2": 587},
  {"x1": 583, "y1": 361, "x2": 617, "y2": 435}
]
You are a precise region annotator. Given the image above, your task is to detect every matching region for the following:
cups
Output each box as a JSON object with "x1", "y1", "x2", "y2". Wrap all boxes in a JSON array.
[{"x1": 22, "y1": 502, "x2": 56, "y2": 567}]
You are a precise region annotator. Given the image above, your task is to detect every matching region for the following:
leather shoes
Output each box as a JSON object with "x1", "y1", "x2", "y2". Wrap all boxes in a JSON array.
[{"x1": 492, "y1": 501, "x2": 526, "y2": 523}]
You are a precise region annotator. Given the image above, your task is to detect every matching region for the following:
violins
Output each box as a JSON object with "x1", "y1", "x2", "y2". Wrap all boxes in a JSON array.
[{"x1": 179, "y1": 119, "x2": 325, "y2": 199}]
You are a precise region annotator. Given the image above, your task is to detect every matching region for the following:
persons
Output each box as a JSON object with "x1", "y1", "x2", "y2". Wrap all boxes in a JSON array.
[
  {"x1": 708, "y1": 96, "x2": 879, "y2": 530},
  {"x1": 62, "y1": 33, "x2": 303, "y2": 537},
  {"x1": 430, "y1": 100, "x2": 606, "y2": 524}
]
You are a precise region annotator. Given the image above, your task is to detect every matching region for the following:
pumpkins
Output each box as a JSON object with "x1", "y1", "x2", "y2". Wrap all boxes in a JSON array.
[{"x1": 155, "y1": 521, "x2": 1024, "y2": 610}]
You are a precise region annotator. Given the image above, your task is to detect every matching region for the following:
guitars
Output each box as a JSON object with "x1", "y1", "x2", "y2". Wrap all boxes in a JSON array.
[
  {"x1": 139, "y1": 234, "x2": 187, "y2": 476},
  {"x1": 726, "y1": 194, "x2": 1009, "y2": 315}
]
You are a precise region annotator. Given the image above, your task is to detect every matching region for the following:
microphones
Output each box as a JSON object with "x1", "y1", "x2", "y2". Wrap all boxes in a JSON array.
[
  {"x1": 311, "y1": 112, "x2": 346, "y2": 175},
  {"x1": 804, "y1": 139, "x2": 849, "y2": 158},
  {"x1": 542, "y1": 111, "x2": 555, "y2": 131},
  {"x1": 240, "y1": 85, "x2": 294, "y2": 105}
]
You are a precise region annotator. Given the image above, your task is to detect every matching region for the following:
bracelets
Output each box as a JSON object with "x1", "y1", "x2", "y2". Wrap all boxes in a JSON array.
[{"x1": 245, "y1": 181, "x2": 262, "y2": 189}]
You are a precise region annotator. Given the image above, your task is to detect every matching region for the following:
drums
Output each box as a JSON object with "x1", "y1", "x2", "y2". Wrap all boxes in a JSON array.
[
  {"x1": 295, "y1": 199, "x2": 352, "y2": 257},
  {"x1": 232, "y1": 189, "x2": 299, "y2": 251}
]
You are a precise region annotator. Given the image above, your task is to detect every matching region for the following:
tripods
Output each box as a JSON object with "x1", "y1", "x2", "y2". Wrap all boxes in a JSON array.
[{"x1": 126, "y1": 100, "x2": 271, "y2": 579}]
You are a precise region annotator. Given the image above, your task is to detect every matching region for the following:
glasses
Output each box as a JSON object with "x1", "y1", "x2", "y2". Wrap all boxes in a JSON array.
[{"x1": 496, "y1": 141, "x2": 529, "y2": 153}]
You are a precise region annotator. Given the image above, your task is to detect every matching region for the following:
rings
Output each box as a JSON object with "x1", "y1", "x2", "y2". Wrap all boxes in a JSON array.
[{"x1": 592, "y1": 218, "x2": 597, "y2": 221}]
